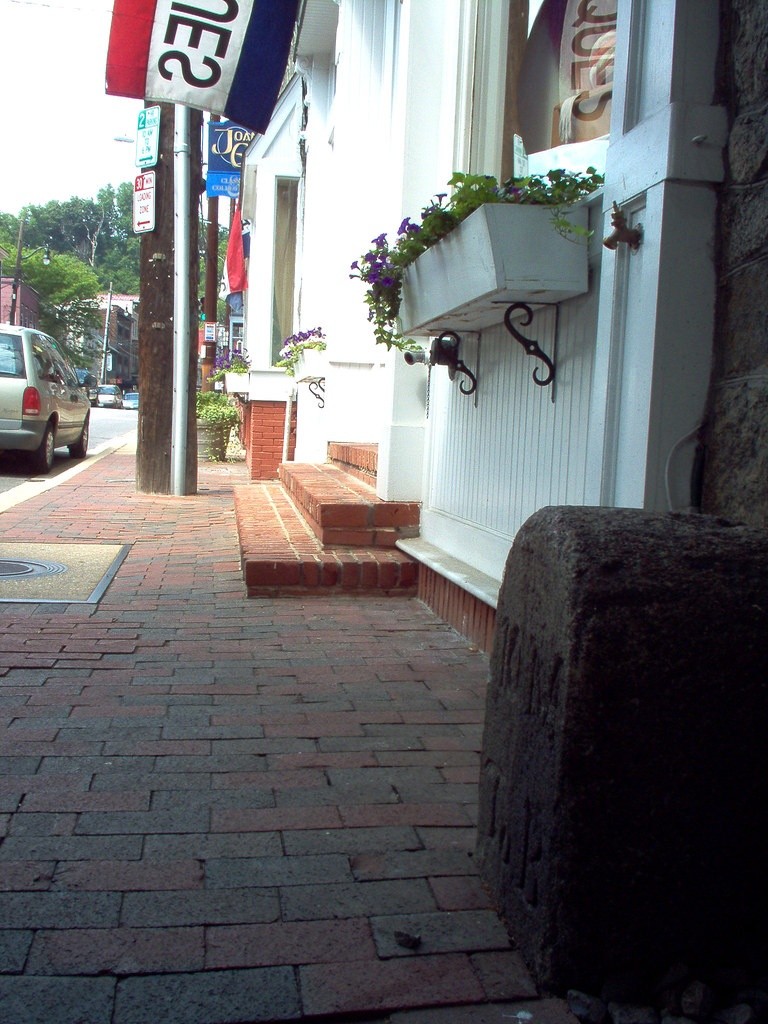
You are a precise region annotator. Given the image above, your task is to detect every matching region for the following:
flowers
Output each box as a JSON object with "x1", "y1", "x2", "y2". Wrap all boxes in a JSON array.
[
  {"x1": 276, "y1": 324, "x2": 327, "y2": 378},
  {"x1": 206, "y1": 346, "x2": 252, "y2": 384},
  {"x1": 348, "y1": 168, "x2": 606, "y2": 370}
]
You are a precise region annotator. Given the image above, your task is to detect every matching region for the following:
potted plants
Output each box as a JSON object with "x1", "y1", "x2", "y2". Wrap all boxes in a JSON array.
[{"x1": 196, "y1": 391, "x2": 246, "y2": 464}]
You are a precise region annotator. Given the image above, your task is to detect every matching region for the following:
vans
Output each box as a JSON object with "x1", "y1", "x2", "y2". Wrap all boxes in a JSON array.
[{"x1": 0, "y1": 323, "x2": 100, "y2": 470}]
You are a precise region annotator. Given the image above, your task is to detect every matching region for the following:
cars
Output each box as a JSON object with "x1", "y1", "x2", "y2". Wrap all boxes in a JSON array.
[
  {"x1": 76, "y1": 370, "x2": 98, "y2": 406},
  {"x1": 122, "y1": 393, "x2": 139, "y2": 410},
  {"x1": 98, "y1": 384, "x2": 123, "y2": 409}
]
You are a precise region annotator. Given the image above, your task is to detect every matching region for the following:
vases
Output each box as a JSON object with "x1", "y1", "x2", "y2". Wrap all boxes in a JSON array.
[
  {"x1": 294, "y1": 347, "x2": 328, "y2": 382},
  {"x1": 224, "y1": 373, "x2": 250, "y2": 393},
  {"x1": 214, "y1": 382, "x2": 224, "y2": 390},
  {"x1": 391, "y1": 199, "x2": 589, "y2": 341}
]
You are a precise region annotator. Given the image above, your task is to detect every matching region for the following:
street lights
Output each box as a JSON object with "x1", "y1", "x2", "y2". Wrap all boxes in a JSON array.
[
  {"x1": 9, "y1": 246, "x2": 52, "y2": 325},
  {"x1": 100, "y1": 304, "x2": 130, "y2": 384}
]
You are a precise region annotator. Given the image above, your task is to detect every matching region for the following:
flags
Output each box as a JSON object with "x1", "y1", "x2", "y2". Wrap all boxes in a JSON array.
[
  {"x1": 218, "y1": 203, "x2": 251, "y2": 313},
  {"x1": 104, "y1": 0, "x2": 303, "y2": 135}
]
